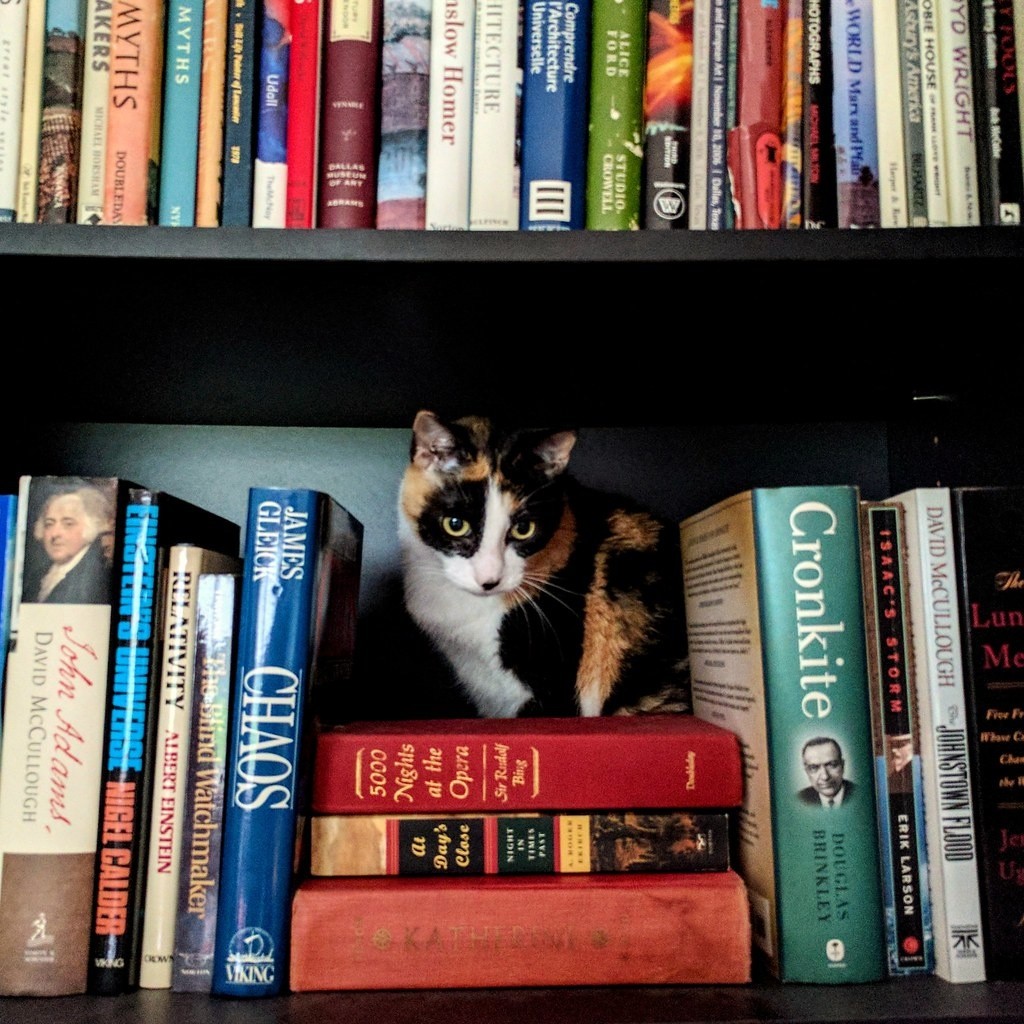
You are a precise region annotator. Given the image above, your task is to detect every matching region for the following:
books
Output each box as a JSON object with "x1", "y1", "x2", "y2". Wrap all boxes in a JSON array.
[
  {"x1": 0, "y1": 0, "x2": 1022, "y2": 236},
  {"x1": 0, "y1": 473, "x2": 1024, "y2": 997}
]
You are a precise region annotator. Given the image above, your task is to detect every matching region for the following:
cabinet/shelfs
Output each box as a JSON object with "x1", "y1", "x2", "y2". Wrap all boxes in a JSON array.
[{"x1": 0, "y1": 0, "x2": 1024, "y2": 1024}]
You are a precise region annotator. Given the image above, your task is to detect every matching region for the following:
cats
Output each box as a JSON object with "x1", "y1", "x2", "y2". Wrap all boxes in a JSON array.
[{"x1": 398, "y1": 410, "x2": 693, "y2": 718}]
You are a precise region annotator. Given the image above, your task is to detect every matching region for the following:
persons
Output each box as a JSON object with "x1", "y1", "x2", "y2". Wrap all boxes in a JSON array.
[
  {"x1": 796, "y1": 737, "x2": 859, "y2": 810},
  {"x1": 21, "y1": 485, "x2": 115, "y2": 604}
]
